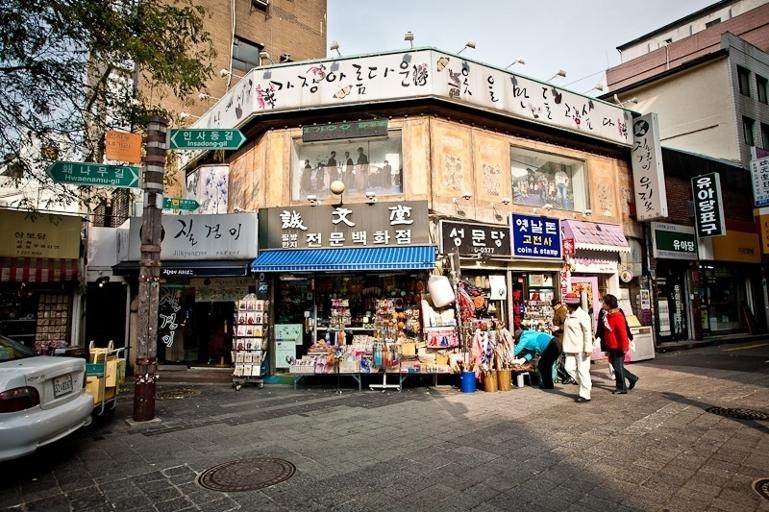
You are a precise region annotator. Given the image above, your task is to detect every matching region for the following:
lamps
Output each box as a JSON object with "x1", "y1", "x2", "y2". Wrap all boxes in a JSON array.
[
  {"x1": 306, "y1": 194, "x2": 322, "y2": 207},
  {"x1": 328, "y1": 179, "x2": 346, "y2": 205},
  {"x1": 173, "y1": 41, "x2": 350, "y2": 124},
  {"x1": 403, "y1": 27, "x2": 640, "y2": 111},
  {"x1": 534, "y1": 203, "x2": 553, "y2": 216},
  {"x1": 452, "y1": 190, "x2": 473, "y2": 205},
  {"x1": 490, "y1": 197, "x2": 513, "y2": 210},
  {"x1": 572, "y1": 209, "x2": 592, "y2": 221},
  {"x1": 477, "y1": 248, "x2": 492, "y2": 265},
  {"x1": 363, "y1": 191, "x2": 378, "y2": 206}
]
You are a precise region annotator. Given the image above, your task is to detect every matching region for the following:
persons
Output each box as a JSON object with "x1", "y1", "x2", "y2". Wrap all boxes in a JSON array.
[
  {"x1": 510, "y1": 326, "x2": 562, "y2": 389},
  {"x1": 555, "y1": 166, "x2": 569, "y2": 208},
  {"x1": 602, "y1": 294, "x2": 639, "y2": 395},
  {"x1": 301, "y1": 147, "x2": 393, "y2": 193},
  {"x1": 562, "y1": 293, "x2": 594, "y2": 402},
  {"x1": 595, "y1": 295, "x2": 633, "y2": 380},
  {"x1": 551, "y1": 299, "x2": 568, "y2": 346}
]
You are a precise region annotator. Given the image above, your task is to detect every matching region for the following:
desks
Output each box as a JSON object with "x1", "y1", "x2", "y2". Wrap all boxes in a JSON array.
[
  {"x1": 284, "y1": 370, "x2": 385, "y2": 393},
  {"x1": 380, "y1": 369, "x2": 455, "y2": 390},
  {"x1": 312, "y1": 326, "x2": 378, "y2": 348}
]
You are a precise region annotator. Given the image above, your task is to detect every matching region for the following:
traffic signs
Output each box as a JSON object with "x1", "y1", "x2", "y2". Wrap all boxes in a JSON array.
[
  {"x1": 166, "y1": 128, "x2": 246, "y2": 152},
  {"x1": 163, "y1": 198, "x2": 202, "y2": 211},
  {"x1": 46, "y1": 161, "x2": 144, "y2": 192}
]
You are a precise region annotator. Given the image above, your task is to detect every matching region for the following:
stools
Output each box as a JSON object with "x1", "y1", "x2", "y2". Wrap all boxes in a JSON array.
[{"x1": 513, "y1": 369, "x2": 533, "y2": 389}]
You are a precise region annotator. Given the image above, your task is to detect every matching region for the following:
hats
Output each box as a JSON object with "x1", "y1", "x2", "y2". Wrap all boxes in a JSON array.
[
  {"x1": 551, "y1": 298, "x2": 561, "y2": 307},
  {"x1": 514, "y1": 329, "x2": 522, "y2": 340},
  {"x1": 564, "y1": 293, "x2": 580, "y2": 304}
]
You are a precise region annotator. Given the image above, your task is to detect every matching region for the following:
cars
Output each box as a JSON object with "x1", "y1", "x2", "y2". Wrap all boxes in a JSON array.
[{"x1": 0, "y1": 335, "x2": 95, "y2": 467}]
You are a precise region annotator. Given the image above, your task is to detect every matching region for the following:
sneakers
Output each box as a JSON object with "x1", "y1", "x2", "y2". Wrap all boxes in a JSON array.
[
  {"x1": 539, "y1": 385, "x2": 554, "y2": 389},
  {"x1": 629, "y1": 376, "x2": 638, "y2": 390},
  {"x1": 612, "y1": 390, "x2": 627, "y2": 394},
  {"x1": 576, "y1": 397, "x2": 591, "y2": 402}
]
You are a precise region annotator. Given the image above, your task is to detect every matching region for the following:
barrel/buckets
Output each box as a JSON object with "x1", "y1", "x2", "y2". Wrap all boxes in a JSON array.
[
  {"x1": 498, "y1": 369, "x2": 512, "y2": 390},
  {"x1": 427, "y1": 274, "x2": 455, "y2": 308},
  {"x1": 484, "y1": 371, "x2": 498, "y2": 392},
  {"x1": 460, "y1": 372, "x2": 475, "y2": 393}
]
